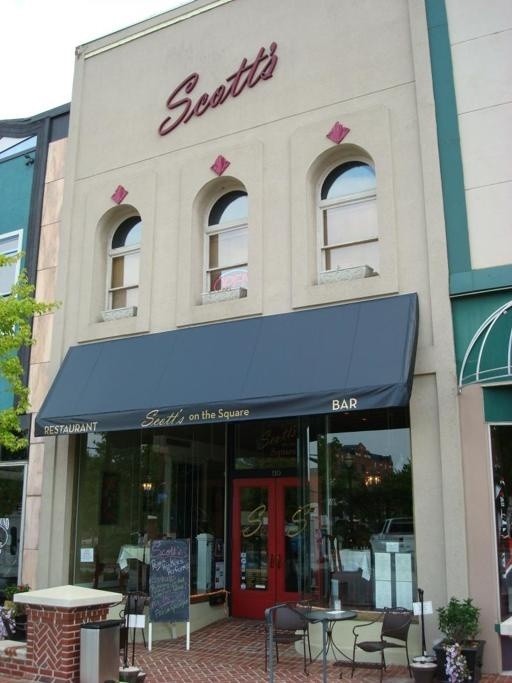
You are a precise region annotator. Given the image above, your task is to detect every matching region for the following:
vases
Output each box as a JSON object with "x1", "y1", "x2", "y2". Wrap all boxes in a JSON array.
[
  {"x1": 410, "y1": 654, "x2": 438, "y2": 682},
  {"x1": 2, "y1": 615, "x2": 29, "y2": 638},
  {"x1": 118, "y1": 665, "x2": 147, "y2": 681}
]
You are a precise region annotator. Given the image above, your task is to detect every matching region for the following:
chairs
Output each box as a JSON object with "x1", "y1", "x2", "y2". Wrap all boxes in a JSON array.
[
  {"x1": 322, "y1": 534, "x2": 348, "y2": 607},
  {"x1": 2, "y1": 613, "x2": 28, "y2": 642},
  {"x1": 264, "y1": 598, "x2": 414, "y2": 683},
  {"x1": 121, "y1": 590, "x2": 148, "y2": 667}
]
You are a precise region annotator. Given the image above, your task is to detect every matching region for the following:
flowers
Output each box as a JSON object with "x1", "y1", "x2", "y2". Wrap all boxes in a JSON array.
[
  {"x1": 1, "y1": 584, "x2": 33, "y2": 639},
  {"x1": 442, "y1": 642, "x2": 473, "y2": 682}
]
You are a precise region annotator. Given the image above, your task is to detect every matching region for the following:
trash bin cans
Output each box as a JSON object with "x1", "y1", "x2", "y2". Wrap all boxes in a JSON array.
[{"x1": 80, "y1": 620, "x2": 120, "y2": 683}]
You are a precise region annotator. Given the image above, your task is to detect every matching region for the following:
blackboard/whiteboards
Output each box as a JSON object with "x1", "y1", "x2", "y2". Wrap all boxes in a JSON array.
[{"x1": 149, "y1": 538, "x2": 190, "y2": 622}]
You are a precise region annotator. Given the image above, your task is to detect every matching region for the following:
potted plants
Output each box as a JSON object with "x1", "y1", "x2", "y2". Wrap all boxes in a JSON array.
[{"x1": 432, "y1": 596, "x2": 486, "y2": 683}]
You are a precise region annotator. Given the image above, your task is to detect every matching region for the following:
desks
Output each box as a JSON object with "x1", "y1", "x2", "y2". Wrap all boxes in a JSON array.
[{"x1": 329, "y1": 549, "x2": 376, "y2": 610}]
